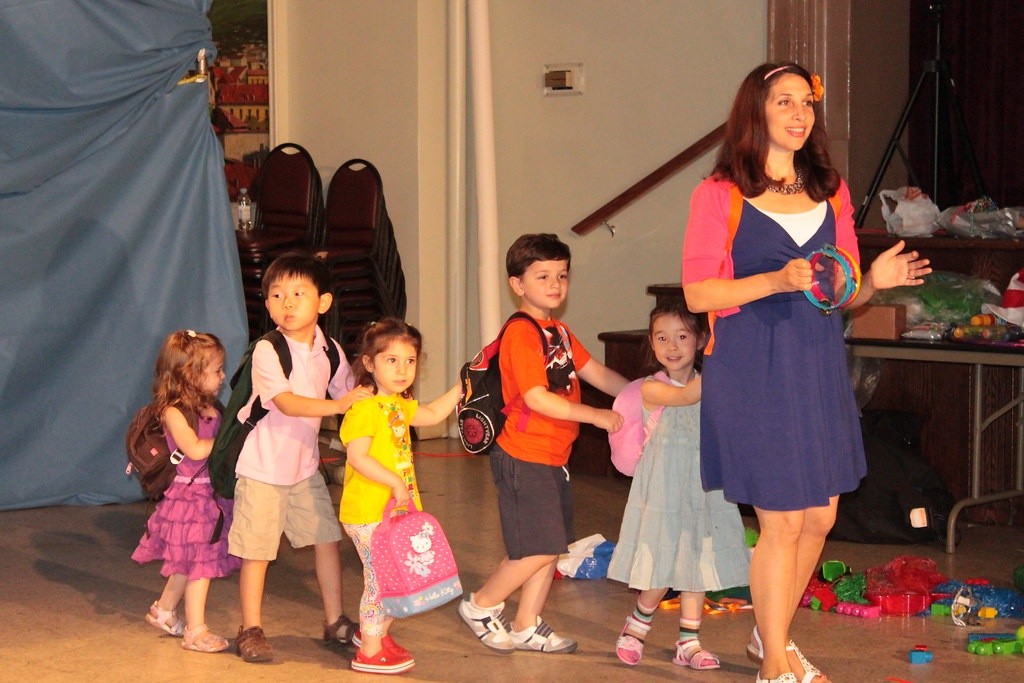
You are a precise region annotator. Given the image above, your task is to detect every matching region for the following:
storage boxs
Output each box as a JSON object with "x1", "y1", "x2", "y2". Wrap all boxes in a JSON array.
[{"x1": 850, "y1": 303, "x2": 906, "y2": 340}]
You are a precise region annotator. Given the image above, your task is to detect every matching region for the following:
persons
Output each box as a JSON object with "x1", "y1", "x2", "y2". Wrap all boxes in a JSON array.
[
  {"x1": 234, "y1": 253, "x2": 367, "y2": 664},
  {"x1": 683, "y1": 61, "x2": 932, "y2": 683},
  {"x1": 130, "y1": 329, "x2": 243, "y2": 653},
  {"x1": 610, "y1": 294, "x2": 753, "y2": 670},
  {"x1": 340, "y1": 317, "x2": 464, "y2": 676},
  {"x1": 456, "y1": 232, "x2": 633, "y2": 654}
]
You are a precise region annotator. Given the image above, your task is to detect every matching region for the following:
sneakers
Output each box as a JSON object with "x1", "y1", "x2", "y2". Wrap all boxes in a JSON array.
[
  {"x1": 508, "y1": 615, "x2": 578, "y2": 653},
  {"x1": 457, "y1": 592, "x2": 516, "y2": 654}
]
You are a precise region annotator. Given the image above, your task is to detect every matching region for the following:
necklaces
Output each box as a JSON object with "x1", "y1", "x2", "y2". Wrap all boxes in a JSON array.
[{"x1": 766, "y1": 166, "x2": 807, "y2": 195}]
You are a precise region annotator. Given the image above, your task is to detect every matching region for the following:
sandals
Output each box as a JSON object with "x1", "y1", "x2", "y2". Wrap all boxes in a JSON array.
[
  {"x1": 235, "y1": 625, "x2": 273, "y2": 662},
  {"x1": 182, "y1": 624, "x2": 228, "y2": 652},
  {"x1": 144, "y1": 601, "x2": 186, "y2": 636},
  {"x1": 672, "y1": 641, "x2": 720, "y2": 670},
  {"x1": 616, "y1": 615, "x2": 651, "y2": 666},
  {"x1": 747, "y1": 625, "x2": 822, "y2": 683},
  {"x1": 323, "y1": 614, "x2": 360, "y2": 649},
  {"x1": 755, "y1": 669, "x2": 798, "y2": 683}
]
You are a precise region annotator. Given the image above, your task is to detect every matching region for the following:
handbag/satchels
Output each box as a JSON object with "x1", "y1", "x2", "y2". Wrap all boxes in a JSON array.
[{"x1": 369, "y1": 496, "x2": 463, "y2": 619}]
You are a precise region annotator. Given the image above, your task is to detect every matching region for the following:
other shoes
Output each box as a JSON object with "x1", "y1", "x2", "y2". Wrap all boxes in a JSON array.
[
  {"x1": 351, "y1": 628, "x2": 411, "y2": 658},
  {"x1": 352, "y1": 644, "x2": 417, "y2": 674}
]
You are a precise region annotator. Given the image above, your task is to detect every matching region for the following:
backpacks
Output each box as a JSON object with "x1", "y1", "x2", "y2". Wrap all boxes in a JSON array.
[
  {"x1": 608, "y1": 369, "x2": 670, "y2": 477},
  {"x1": 126, "y1": 401, "x2": 200, "y2": 501},
  {"x1": 454, "y1": 311, "x2": 572, "y2": 456},
  {"x1": 206, "y1": 329, "x2": 341, "y2": 500}
]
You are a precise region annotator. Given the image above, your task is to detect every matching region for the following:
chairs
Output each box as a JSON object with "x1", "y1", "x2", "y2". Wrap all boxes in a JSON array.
[{"x1": 227, "y1": 144, "x2": 419, "y2": 444}]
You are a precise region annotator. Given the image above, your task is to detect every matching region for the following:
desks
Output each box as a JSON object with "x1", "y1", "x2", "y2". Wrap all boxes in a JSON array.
[{"x1": 844, "y1": 339, "x2": 1024, "y2": 553}]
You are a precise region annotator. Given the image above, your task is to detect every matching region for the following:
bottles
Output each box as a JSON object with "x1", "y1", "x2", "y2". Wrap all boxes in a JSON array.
[{"x1": 238, "y1": 189, "x2": 251, "y2": 231}]
